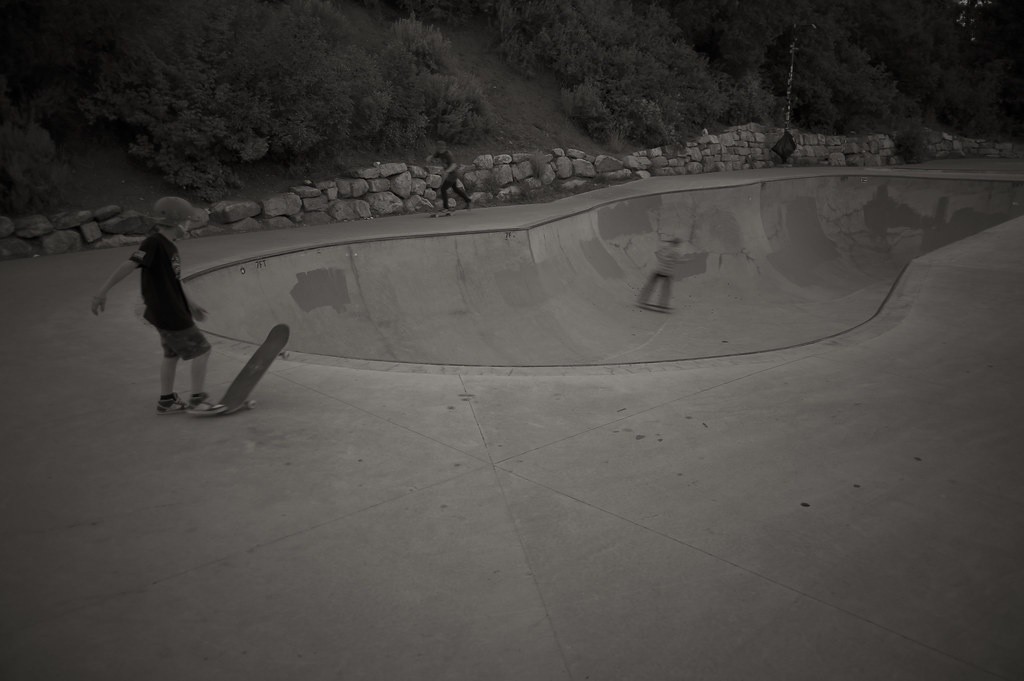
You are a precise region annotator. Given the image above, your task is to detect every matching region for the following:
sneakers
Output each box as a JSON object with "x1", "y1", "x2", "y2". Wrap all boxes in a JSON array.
[
  {"x1": 156, "y1": 392, "x2": 188, "y2": 415},
  {"x1": 186, "y1": 393, "x2": 227, "y2": 415}
]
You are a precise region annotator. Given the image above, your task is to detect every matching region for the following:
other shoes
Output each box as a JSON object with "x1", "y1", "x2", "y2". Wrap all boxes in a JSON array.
[
  {"x1": 437, "y1": 208, "x2": 450, "y2": 216},
  {"x1": 464, "y1": 200, "x2": 474, "y2": 210}
]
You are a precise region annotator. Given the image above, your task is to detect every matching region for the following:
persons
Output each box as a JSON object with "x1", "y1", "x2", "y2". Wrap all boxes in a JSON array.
[
  {"x1": 636, "y1": 236, "x2": 682, "y2": 309},
  {"x1": 92, "y1": 196, "x2": 229, "y2": 416},
  {"x1": 427, "y1": 139, "x2": 472, "y2": 213}
]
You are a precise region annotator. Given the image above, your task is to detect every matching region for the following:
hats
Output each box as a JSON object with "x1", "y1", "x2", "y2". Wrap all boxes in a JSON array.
[{"x1": 151, "y1": 197, "x2": 194, "y2": 226}]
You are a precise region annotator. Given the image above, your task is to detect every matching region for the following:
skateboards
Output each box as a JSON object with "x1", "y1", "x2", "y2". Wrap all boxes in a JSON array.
[
  {"x1": 635, "y1": 301, "x2": 675, "y2": 310},
  {"x1": 217, "y1": 324, "x2": 291, "y2": 415},
  {"x1": 428, "y1": 207, "x2": 457, "y2": 217}
]
